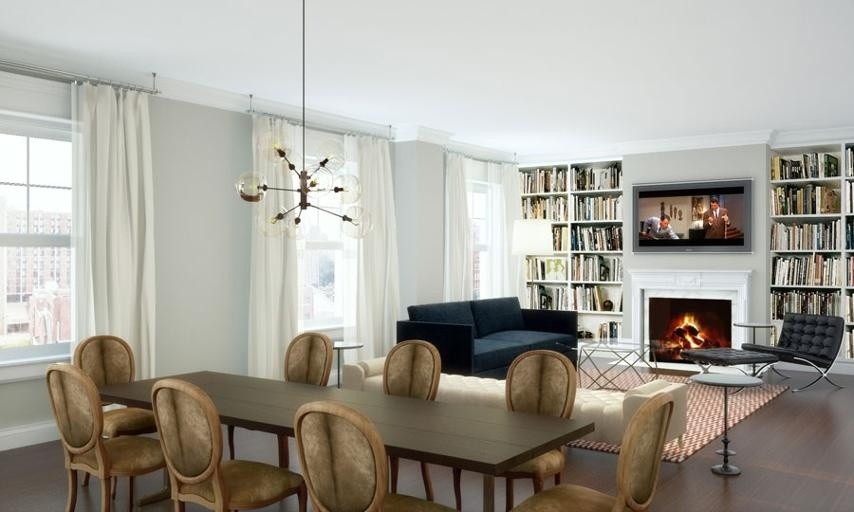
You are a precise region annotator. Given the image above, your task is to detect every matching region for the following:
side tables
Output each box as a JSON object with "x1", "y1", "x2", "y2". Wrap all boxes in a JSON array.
[{"x1": 734, "y1": 322, "x2": 775, "y2": 393}]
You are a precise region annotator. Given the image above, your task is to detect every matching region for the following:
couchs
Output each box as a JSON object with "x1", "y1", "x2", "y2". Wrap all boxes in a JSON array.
[{"x1": 395, "y1": 309, "x2": 579, "y2": 381}]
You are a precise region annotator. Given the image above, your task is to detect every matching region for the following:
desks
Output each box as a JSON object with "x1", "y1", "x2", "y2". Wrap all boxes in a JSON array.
[
  {"x1": 93, "y1": 369, "x2": 593, "y2": 512},
  {"x1": 327, "y1": 340, "x2": 364, "y2": 389}
]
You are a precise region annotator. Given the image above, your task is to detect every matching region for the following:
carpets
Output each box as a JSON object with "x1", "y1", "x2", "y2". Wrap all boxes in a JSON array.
[{"x1": 568, "y1": 362, "x2": 789, "y2": 463}]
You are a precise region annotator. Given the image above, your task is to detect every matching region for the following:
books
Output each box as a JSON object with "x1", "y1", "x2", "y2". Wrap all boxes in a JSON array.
[
  {"x1": 770, "y1": 146, "x2": 854, "y2": 359},
  {"x1": 519, "y1": 162, "x2": 624, "y2": 338}
]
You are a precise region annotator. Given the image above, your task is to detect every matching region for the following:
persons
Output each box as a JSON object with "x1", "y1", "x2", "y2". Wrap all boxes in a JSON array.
[
  {"x1": 703, "y1": 198, "x2": 731, "y2": 239},
  {"x1": 642, "y1": 215, "x2": 679, "y2": 240}
]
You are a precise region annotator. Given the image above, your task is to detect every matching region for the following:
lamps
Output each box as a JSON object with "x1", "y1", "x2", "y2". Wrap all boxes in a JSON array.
[
  {"x1": 511, "y1": 218, "x2": 553, "y2": 309},
  {"x1": 236, "y1": 0, "x2": 372, "y2": 240}
]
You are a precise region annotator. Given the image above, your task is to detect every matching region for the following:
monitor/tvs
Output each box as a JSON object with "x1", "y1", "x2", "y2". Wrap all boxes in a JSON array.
[{"x1": 631, "y1": 181, "x2": 752, "y2": 253}]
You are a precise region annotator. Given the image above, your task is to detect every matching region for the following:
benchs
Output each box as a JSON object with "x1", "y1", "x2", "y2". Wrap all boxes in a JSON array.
[
  {"x1": 343, "y1": 356, "x2": 686, "y2": 448},
  {"x1": 742, "y1": 311, "x2": 845, "y2": 391},
  {"x1": 679, "y1": 348, "x2": 778, "y2": 393}
]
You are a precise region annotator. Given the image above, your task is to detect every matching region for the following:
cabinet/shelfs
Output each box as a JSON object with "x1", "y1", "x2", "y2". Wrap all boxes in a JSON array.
[
  {"x1": 768, "y1": 139, "x2": 854, "y2": 373},
  {"x1": 517, "y1": 158, "x2": 623, "y2": 340}
]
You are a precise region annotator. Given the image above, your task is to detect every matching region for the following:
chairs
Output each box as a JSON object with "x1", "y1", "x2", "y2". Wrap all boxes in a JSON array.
[
  {"x1": 480, "y1": 349, "x2": 578, "y2": 512},
  {"x1": 502, "y1": 392, "x2": 675, "y2": 512}
]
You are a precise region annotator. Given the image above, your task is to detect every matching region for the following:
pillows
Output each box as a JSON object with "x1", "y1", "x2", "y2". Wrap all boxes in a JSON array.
[
  {"x1": 406, "y1": 301, "x2": 483, "y2": 338},
  {"x1": 470, "y1": 296, "x2": 525, "y2": 335}
]
